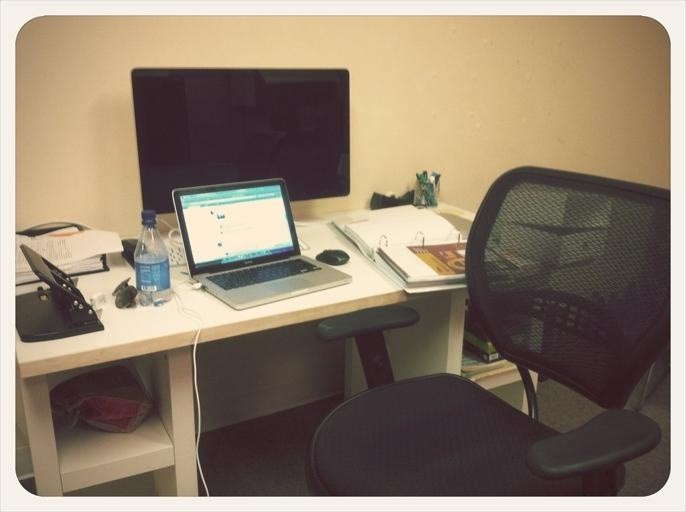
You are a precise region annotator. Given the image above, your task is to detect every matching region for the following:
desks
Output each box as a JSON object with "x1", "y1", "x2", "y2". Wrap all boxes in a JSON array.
[{"x1": 16, "y1": 201, "x2": 540, "y2": 496}]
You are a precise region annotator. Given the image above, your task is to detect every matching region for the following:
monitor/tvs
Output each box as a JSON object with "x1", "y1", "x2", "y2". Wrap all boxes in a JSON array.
[{"x1": 130, "y1": 67, "x2": 351, "y2": 213}]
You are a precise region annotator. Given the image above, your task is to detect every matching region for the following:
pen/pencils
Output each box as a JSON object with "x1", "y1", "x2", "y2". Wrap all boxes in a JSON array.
[{"x1": 416, "y1": 171, "x2": 441, "y2": 206}]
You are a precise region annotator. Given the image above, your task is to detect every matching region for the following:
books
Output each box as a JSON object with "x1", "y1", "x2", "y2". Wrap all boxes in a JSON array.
[
  {"x1": 332, "y1": 202, "x2": 519, "y2": 297},
  {"x1": 460, "y1": 346, "x2": 519, "y2": 383}
]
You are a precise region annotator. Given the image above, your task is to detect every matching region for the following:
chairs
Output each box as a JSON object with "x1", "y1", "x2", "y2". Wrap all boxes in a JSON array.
[{"x1": 310, "y1": 166, "x2": 670, "y2": 496}]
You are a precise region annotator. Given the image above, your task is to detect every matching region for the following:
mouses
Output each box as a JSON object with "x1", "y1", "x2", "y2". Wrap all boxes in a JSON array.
[{"x1": 316, "y1": 249, "x2": 350, "y2": 266}]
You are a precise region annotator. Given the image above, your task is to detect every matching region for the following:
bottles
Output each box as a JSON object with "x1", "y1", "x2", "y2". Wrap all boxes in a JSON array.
[{"x1": 134, "y1": 211, "x2": 172, "y2": 307}]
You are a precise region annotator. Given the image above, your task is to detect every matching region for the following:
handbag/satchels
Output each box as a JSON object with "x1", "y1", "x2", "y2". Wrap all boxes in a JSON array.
[{"x1": 50, "y1": 366, "x2": 149, "y2": 432}]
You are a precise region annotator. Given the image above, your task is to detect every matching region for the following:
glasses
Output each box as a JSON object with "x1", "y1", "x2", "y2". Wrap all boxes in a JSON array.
[{"x1": 112, "y1": 278, "x2": 139, "y2": 309}]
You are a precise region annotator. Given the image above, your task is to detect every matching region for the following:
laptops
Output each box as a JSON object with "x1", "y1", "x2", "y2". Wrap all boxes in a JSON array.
[{"x1": 172, "y1": 177, "x2": 352, "y2": 311}]
